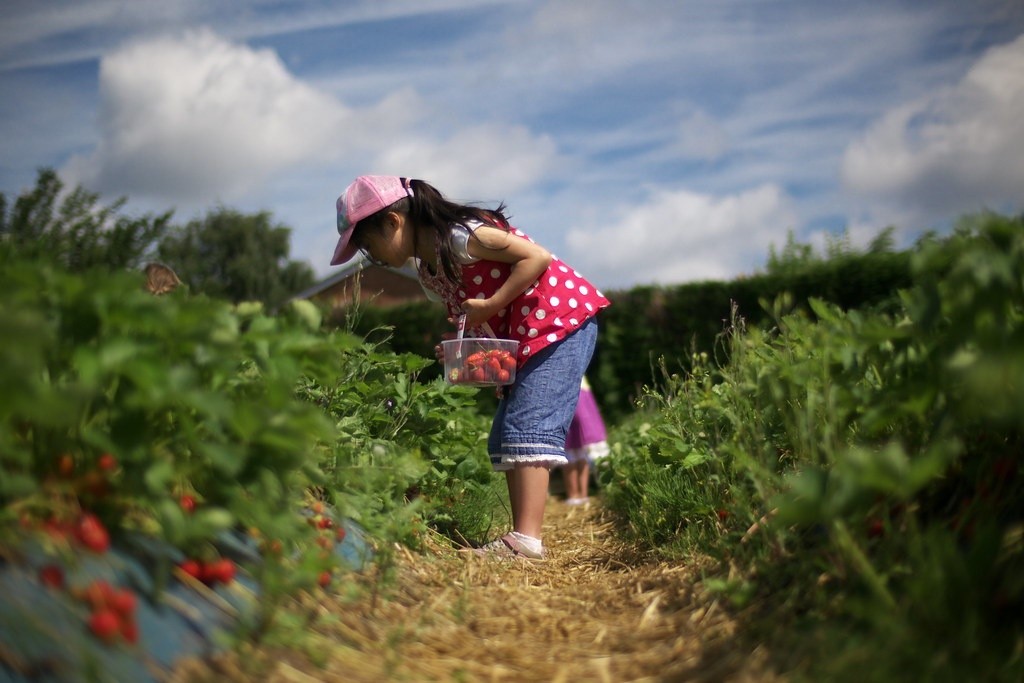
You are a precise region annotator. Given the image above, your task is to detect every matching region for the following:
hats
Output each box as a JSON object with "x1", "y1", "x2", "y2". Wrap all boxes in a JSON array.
[{"x1": 331, "y1": 175, "x2": 412, "y2": 265}]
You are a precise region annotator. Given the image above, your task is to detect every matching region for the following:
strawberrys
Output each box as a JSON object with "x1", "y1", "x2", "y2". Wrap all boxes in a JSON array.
[
  {"x1": 865, "y1": 519, "x2": 883, "y2": 540},
  {"x1": 15, "y1": 453, "x2": 345, "y2": 644},
  {"x1": 450, "y1": 348, "x2": 517, "y2": 383},
  {"x1": 720, "y1": 510, "x2": 726, "y2": 521}
]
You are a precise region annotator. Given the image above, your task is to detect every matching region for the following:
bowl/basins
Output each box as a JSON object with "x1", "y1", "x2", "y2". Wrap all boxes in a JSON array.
[{"x1": 441, "y1": 338, "x2": 520, "y2": 387}]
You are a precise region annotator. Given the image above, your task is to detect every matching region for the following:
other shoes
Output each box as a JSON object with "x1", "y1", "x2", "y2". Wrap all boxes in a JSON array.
[{"x1": 480, "y1": 536, "x2": 542, "y2": 561}]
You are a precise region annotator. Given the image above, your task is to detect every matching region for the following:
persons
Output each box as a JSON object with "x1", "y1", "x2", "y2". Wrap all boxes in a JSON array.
[
  {"x1": 561, "y1": 374, "x2": 610, "y2": 506},
  {"x1": 144, "y1": 262, "x2": 181, "y2": 296},
  {"x1": 330, "y1": 174, "x2": 609, "y2": 562}
]
